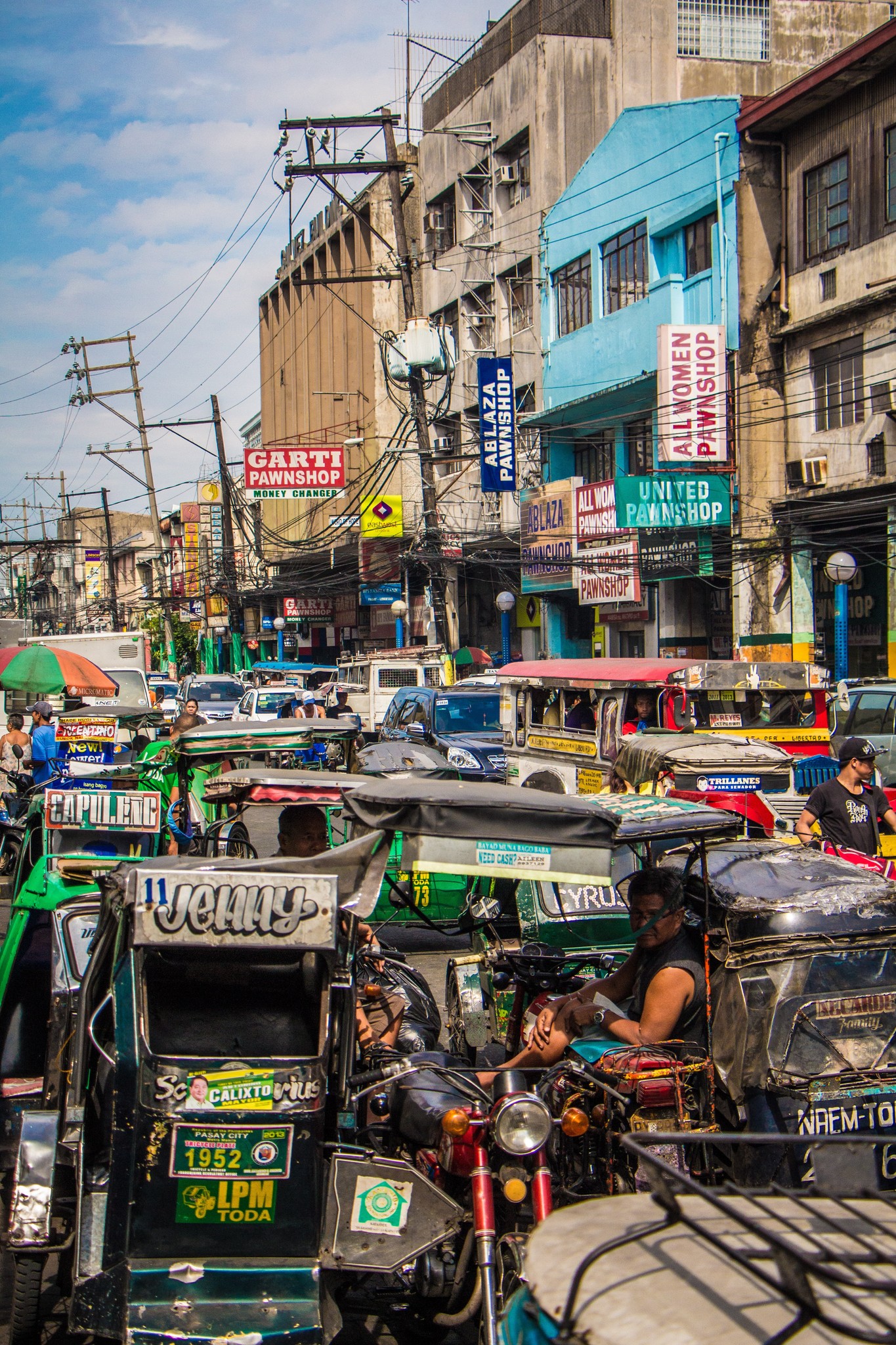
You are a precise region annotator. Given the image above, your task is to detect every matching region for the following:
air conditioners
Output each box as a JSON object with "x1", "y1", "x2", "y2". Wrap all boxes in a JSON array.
[
  {"x1": 423, "y1": 213, "x2": 439, "y2": 234},
  {"x1": 495, "y1": 165, "x2": 515, "y2": 184},
  {"x1": 870, "y1": 380, "x2": 896, "y2": 414},
  {"x1": 785, "y1": 456, "x2": 826, "y2": 489},
  {"x1": 434, "y1": 437, "x2": 452, "y2": 452}
]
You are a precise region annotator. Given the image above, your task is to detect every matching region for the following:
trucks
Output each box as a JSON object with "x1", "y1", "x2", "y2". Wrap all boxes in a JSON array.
[
  {"x1": 0, "y1": 631, "x2": 166, "y2": 751},
  {"x1": 320, "y1": 644, "x2": 447, "y2": 734},
  {"x1": 242, "y1": 661, "x2": 338, "y2": 707}
]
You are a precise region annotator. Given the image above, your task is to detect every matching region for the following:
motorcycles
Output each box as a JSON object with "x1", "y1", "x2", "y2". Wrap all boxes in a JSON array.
[{"x1": 0, "y1": 704, "x2": 896, "y2": 1345}]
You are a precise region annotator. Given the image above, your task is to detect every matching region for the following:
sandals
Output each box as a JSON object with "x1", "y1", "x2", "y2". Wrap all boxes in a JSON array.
[{"x1": 360, "y1": 1041, "x2": 399, "y2": 1070}]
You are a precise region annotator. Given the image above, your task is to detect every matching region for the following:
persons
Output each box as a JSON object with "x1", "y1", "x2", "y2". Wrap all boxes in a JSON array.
[
  {"x1": 295, "y1": 690, "x2": 327, "y2": 771},
  {"x1": 202, "y1": 662, "x2": 204, "y2": 667},
  {"x1": 0, "y1": 713, "x2": 33, "y2": 809},
  {"x1": 168, "y1": 663, "x2": 177, "y2": 678},
  {"x1": 735, "y1": 689, "x2": 771, "y2": 728},
  {"x1": 622, "y1": 689, "x2": 655, "y2": 736},
  {"x1": 268, "y1": 797, "x2": 407, "y2": 1137},
  {"x1": 146, "y1": 678, "x2": 160, "y2": 708},
  {"x1": 22, "y1": 701, "x2": 62, "y2": 793},
  {"x1": 185, "y1": 698, "x2": 208, "y2": 726},
  {"x1": 326, "y1": 692, "x2": 366, "y2": 749},
  {"x1": 470, "y1": 865, "x2": 708, "y2": 1091},
  {"x1": 524, "y1": 687, "x2": 599, "y2": 736},
  {"x1": 795, "y1": 737, "x2": 896, "y2": 854},
  {"x1": 129, "y1": 713, "x2": 236, "y2": 863}
]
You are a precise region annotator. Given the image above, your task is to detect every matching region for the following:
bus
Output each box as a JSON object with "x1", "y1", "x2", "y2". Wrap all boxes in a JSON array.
[{"x1": 496, "y1": 658, "x2": 850, "y2": 794}]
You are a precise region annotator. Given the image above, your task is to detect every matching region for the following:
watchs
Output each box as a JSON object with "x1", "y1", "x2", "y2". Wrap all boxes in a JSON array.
[{"x1": 594, "y1": 1007, "x2": 610, "y2": 1027}]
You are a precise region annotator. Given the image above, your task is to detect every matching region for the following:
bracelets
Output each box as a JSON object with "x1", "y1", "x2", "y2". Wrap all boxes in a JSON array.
[{"x1": 567, "y1": 992, "x2": 572, "y2": 1001}]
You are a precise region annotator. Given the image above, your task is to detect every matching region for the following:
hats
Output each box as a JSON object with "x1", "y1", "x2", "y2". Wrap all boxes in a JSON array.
[
  {"x1": 25, "y1": 701, "x2": 53, "y2": 717},
  {"x1": 302, "y1": 691, "x2": 315, "y2": 705},
  {"x1": 838, "y1": 737, "x2": 888, "y2": 761}
]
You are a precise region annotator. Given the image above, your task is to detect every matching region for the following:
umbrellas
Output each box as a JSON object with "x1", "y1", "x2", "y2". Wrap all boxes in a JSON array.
[
  {"x1": 450, "y1": 646, "x2": 492, "y2": 678},
  {"x1": 0, "y1": 644, "x2": 122, "y2": 706}
]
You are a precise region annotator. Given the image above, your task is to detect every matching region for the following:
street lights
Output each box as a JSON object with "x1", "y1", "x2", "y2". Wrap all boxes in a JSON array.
[
  {"x1": 341, "y1": 434, "x2": 447, "y2": 651},
  {"x1": 389, "y1": 599, "x2": 409, "y2": 648},
  {"x1": 272, "y1": 617, "x2": 286, "y2": 662},
  {"x1": 213, "y1": 627, "x2": 227, "y2": 674},
  {"x1": 494, "y1": 591, "x2": 516, "y2": 667},
  {"x1": 823, "y1": 552, "x2": 860, "y2": 691}
]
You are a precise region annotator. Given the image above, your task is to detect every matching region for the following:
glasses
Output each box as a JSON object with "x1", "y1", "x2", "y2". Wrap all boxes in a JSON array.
[{"x1": 628, "y1": 909, "x2": 676, "y2": 922}]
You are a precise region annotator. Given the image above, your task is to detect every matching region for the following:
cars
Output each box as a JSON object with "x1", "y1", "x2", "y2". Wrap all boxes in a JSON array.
[
  {"x1": 453, "y1": 668, "x2": 500, "y2": 685},
  {"x1": 147, "y1": 676, "x2": 181, "y2": 726},
  {"x1": 232, "y1": 685, "x2": 308, "y2": 761}
]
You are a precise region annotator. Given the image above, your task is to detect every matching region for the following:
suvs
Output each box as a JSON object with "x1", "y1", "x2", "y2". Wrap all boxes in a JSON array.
[
  {"x1": 174, "y1": 672, "x2": 247, "y2": 724},
  {"x1": 801, "y1": 681, "x2": 896, "y2": 789},
  {"x1": 376, "y1": 684, "x2": 508, "y2": 785}
]
